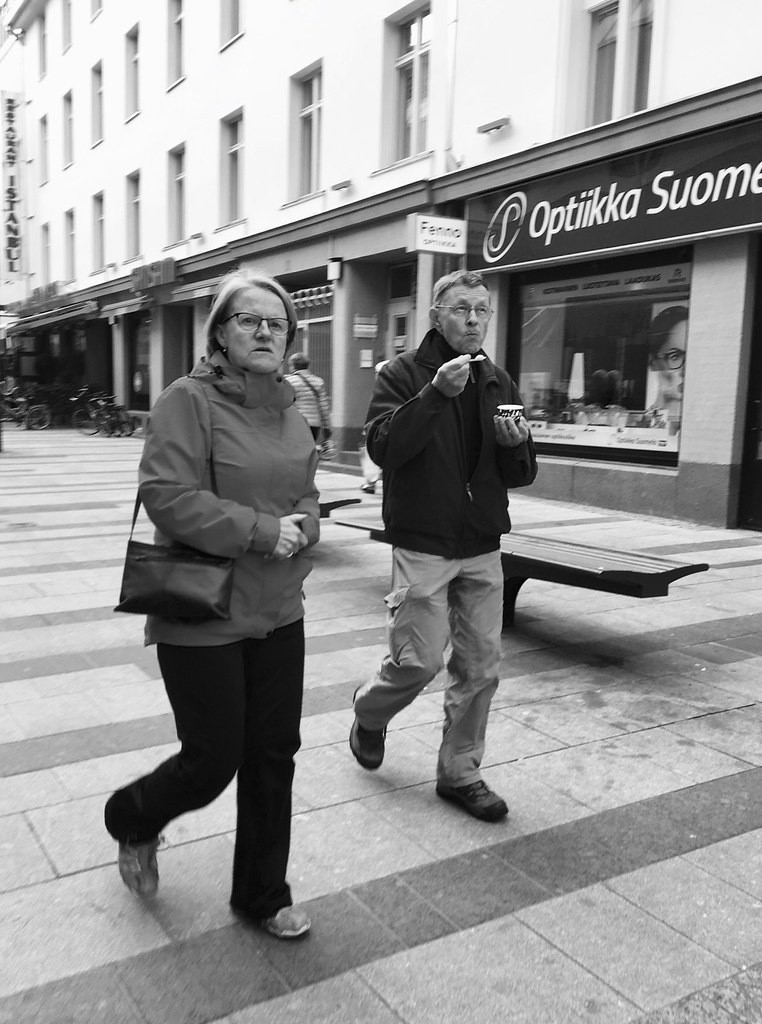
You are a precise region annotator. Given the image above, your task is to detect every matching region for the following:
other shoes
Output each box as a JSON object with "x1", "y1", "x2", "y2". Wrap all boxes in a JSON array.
[{"x1": 361, "y1": 484, "x2": 375, "y2": 494}]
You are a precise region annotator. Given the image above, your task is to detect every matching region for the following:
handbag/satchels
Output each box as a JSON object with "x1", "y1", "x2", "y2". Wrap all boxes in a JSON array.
[{"x1": 112, "y1": 540, "x2": 234, "y2": 622}]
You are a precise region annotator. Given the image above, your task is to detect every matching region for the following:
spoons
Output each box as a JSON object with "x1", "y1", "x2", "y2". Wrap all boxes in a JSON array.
[{"x1": 469, "y1": 357, "x2": 487, "y2": 362}]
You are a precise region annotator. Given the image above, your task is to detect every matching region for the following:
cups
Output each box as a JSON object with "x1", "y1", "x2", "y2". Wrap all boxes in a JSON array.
[{"x1": 497, "y1": 404, "x2": 524, "y2": 423}]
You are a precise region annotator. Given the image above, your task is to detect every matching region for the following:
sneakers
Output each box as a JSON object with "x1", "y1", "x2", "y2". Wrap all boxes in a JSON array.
[
  {"x1": 257, "y1": 905, "x2": 311, "y2": 938},
  {"x1": 435, "y1": 780, "x2": 508, "y2": 822},
  {"x1": 349, "y1": 684, "x2": 387, "y2": 770},
  {"x1": 118, "y1": 836, "x2": 159, "y2": 896}
]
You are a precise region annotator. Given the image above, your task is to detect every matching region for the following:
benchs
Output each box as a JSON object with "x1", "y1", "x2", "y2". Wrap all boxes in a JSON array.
[{"x1": 337, "y1": 517, "x2": 711, "y2": 631}]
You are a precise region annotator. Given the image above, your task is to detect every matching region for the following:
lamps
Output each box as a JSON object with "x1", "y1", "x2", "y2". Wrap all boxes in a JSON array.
[
  {"x1": 332, "y1": 180, "x2": 351, "y2": 192},
  {"x1": 186, "y1": 232, "x2": 204, "y2": 240},
  {"x1": 478, "y1": 115, "x2": 510, "y2": 135},
  {"x1": 327, "y1": 256, "x2": 343, "y2": 282},
  {"x1": 106, "y1": 262, "x2": 115, "y2": 268}
]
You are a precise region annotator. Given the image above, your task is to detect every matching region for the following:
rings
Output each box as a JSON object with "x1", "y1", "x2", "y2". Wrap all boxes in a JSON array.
[{"x1": 287, "y1": 552, "x2": 293, "y2": 558}]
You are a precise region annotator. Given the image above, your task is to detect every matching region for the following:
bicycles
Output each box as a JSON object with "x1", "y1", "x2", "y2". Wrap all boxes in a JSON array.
[
  {"x1": 69, "y1": 385, "x2": 135, "y2": 439},
  {"x1": 0, "y1": 380, "x2": 52, "y2": 430}
]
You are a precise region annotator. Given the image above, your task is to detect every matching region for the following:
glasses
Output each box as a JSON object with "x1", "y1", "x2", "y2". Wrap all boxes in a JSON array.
[
  {"x1": 655, "y1": 349, "x2": 686, "y2": 370},
  {"x1": 219, "y1": 312, "x2": 292, "y2": 335},
  {"x1": 435, "y1": 304, "x2": 493, "y2": 318}
]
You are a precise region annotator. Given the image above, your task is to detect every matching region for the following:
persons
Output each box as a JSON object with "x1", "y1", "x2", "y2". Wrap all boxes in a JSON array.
[
  {"x1": 649, "y1": 306, "x2": 688, "y2": 415},
  {"x1": 283, "y1": 352, "x2": 332, "y2": 442},
  {"x1": 588, "y1": 369, "x2": 622, "y2": 408},
  {"x1": 348, "y1": 270, "x2": 540, "y2": 821},
  {"x1": 104, "y1": 276, "x2": 321, "y2": 937},
  {"x1": 358, "y1": 360, "x2": 391, "y2": 493}
]
